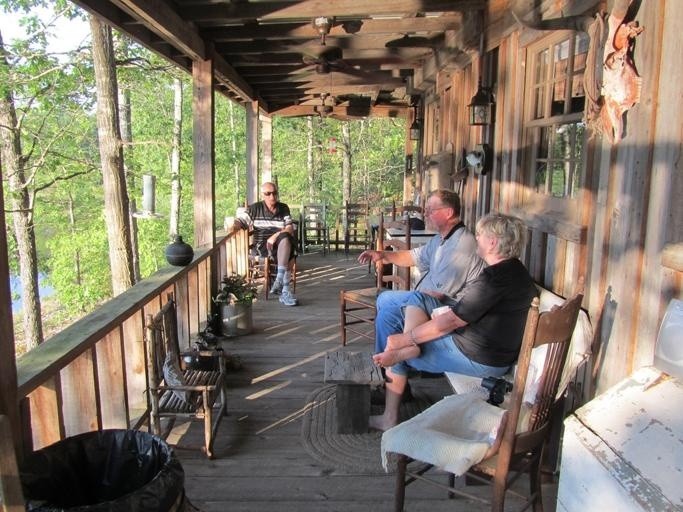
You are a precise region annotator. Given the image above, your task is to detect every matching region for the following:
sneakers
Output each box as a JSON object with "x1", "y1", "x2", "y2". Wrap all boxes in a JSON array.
[
  {"x1": 269, "y1": 280, "x2": 283, "y2": 295},
  {"x1": 279, "y1": 292, "x2": 297, "y2": 305},
  {"x1": 370, "y1": 381, "x2": 412, "y2": 404}
]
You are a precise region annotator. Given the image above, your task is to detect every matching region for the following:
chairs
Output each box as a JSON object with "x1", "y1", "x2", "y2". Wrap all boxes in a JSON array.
[
  {"x1": 146, "y1": 292, "x2": 227, "y2": 460},
  {"x1": 340, "y1": 211, "x2": 411, "y2": 348},
  {"x1": 381, "y1": 292, "x2": 584, "y2": 512},
  {"x1": 392, "y1": 198, "x2": 425, "y2": 224},
  {"x1": 245, "y1": 227, "x2": 298, "y2": 300},
  {"x1": 300, "y1": 199, "x2": 330, "y2": 254},
  {"x1": 345, "y1": 200, "x2": 373, "y2": 253}
]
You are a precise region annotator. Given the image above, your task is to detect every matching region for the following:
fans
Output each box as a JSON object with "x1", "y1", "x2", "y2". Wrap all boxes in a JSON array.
[
  {"x1": 281, "y1": 94, "x2": 351, "y2": 122},
  {"x1": 273, "y1": 17, "x2": 371, "y2": 81}
]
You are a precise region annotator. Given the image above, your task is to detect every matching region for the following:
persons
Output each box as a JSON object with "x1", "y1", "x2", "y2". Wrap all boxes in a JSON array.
[
  {"x1": 223, "y1": 181, "x2": 301, "y2": 308},
  {"x1": 367, "y1": 207, "x2": 541, "y2": 433},
  {"x1": 356, "y1": 188, "x2": 490, "y2": 407}
]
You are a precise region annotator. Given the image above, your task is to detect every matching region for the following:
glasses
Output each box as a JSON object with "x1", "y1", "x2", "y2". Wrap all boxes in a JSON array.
[
  {"x1": 263, "y1": 191, "x2": 278, "y2": 196},
  {"x1": 423, "y1": 207, "x2": 442, "y2": 214}
]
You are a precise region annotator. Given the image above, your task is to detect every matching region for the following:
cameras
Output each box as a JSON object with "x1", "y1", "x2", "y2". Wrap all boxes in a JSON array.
[{"x1": 480, "y1": 375, "x2": 513, "y2": 406}]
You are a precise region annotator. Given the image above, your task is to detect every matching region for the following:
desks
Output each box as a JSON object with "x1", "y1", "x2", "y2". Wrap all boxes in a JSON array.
[{"x1": 385, "y1": 225, "x2": 439, "y2": 291}]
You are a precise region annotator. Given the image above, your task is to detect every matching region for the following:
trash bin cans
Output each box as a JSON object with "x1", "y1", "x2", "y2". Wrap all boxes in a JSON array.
[{"x1": 34, "y1": 429, "x2": 187, "y2": 511}]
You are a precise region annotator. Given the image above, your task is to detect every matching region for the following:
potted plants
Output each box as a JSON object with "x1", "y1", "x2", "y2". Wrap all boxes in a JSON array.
[{"x1": 212, "y1": 271, "x2": 258, "y2": 338}]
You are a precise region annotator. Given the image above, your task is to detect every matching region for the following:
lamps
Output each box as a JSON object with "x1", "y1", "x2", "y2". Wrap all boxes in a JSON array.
[
  {"x1": 409, "y1": 118, "x2": 421, "y2": 141},
  {"x1": 466, "y1": 74, "x2": 495, "y2": 126}
]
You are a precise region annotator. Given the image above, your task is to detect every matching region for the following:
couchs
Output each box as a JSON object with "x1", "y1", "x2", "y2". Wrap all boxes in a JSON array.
[{"x1": 444, "y1": 283, "x2": 594, "y2": 487}]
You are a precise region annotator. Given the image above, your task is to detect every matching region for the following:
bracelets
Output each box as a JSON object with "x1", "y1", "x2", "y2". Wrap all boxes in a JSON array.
[{"x1": 408, "y1": 327, "x2": 418, "y2": 346}]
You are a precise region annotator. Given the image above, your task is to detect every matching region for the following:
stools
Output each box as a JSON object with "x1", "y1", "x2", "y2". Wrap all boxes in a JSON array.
[{"x1": 324, "y1": 349, "x2": 383, "y2": 433}]
